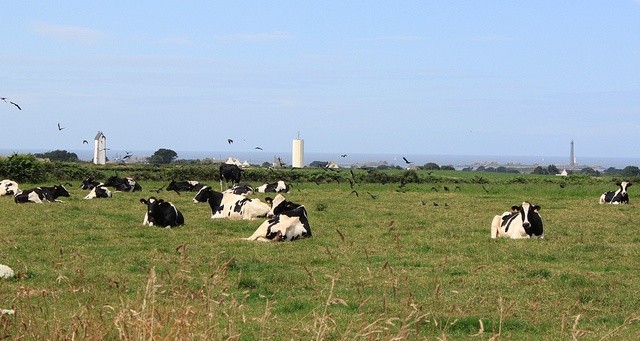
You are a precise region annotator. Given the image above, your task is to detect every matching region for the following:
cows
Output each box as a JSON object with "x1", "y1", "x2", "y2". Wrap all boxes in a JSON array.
[
  {"x1": 600, "y1": 181, "x2": 632, "y2": 204},
  {"x1": 103, "y1": 175, "x2": 141, "y2": 192},
  {"x1": 80, "y1": 177, "x2": 104, "y2": 189},
  {"x1": 140, "y1": 196, "x2": 184, "y2": 228},
  {"x1": 0, "y1": 179, "x2": 18, "y2": 196},
  {"x1": 491, "y1": 201, "x2": 545, "y2": 239},
  {"x1": 83, "y1": 183, "x2": 112, "y2": 199},
  {"x1": 224, "y1": 183, "x2": 254, "y2": 195},
  {"x1": 241, "y1": 193, "x2": 311, "y2": 241},
  {"x1": 255, "y1": 180, "x2": 293, "y2": 192},
  {"x1": 166, "y1": 176, "x2": 205, "y2": 194},
  {"x1": 219, "y1": 163, "x2": 244, "y2": 190},
  {"x1": 15, "y1": 184, "x2": 70, "y2": 202},
  {"x1": 192, "y1": 185, "x2": 272, "y2": 219}
]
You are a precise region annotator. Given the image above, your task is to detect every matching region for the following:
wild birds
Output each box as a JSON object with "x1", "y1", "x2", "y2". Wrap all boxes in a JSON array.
[
  {"x1": 10, "y1": 100, "x2": 22, "y2": 112},
  {"x1": 309, "y1": 154, "x2": 490, "y2": 207},
  {"x1": 254, "y1": 146, "x2": 263, "y2": 150},
  {"x1": 0, "y1": 97, "x2": 9, "y2": 104},
  {"x1": 58, "y1": 123, "x2": 65, "y2": 131},
  {"x1": 228, "y1": 138, "x2": 233, "y2": 143},
  {"x1": 83, "y1": 139, "x2": 89, "y2": 144}
]
